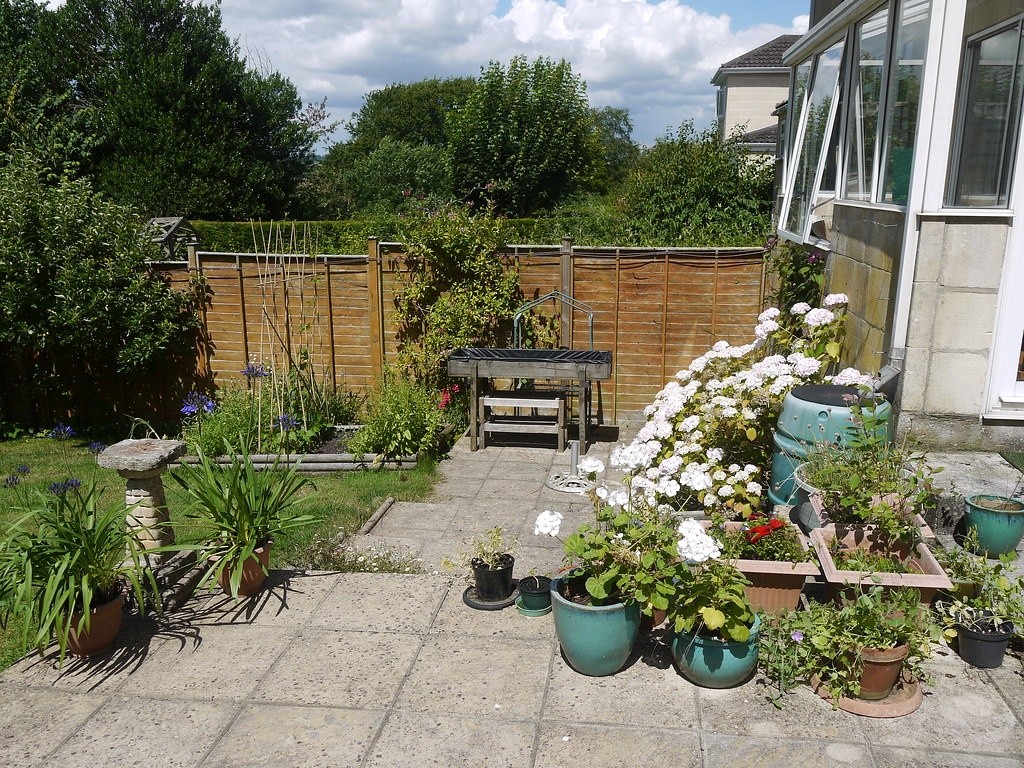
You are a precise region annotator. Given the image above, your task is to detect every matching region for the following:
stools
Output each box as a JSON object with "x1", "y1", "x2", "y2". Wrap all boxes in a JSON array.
[{"x1": 479, "y1": 388, "x2": 569, "y2": 453}]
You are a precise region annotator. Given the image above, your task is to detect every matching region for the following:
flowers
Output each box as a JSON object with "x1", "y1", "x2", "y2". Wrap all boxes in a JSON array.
[{"x1": 535, "y1": 289, "x2": 877, "y2": 558}]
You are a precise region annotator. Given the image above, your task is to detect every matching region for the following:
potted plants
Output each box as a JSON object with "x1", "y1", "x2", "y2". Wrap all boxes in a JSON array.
[
  {"x1": 139, "y1": 424, "x2": 329, "y2": 599},
  {"x1": 447, "y1": 367, "x2": 1024, "y2": 711},
  {"x1": 0, "y1": 451, "x2": 164, "y2": 676}
]
